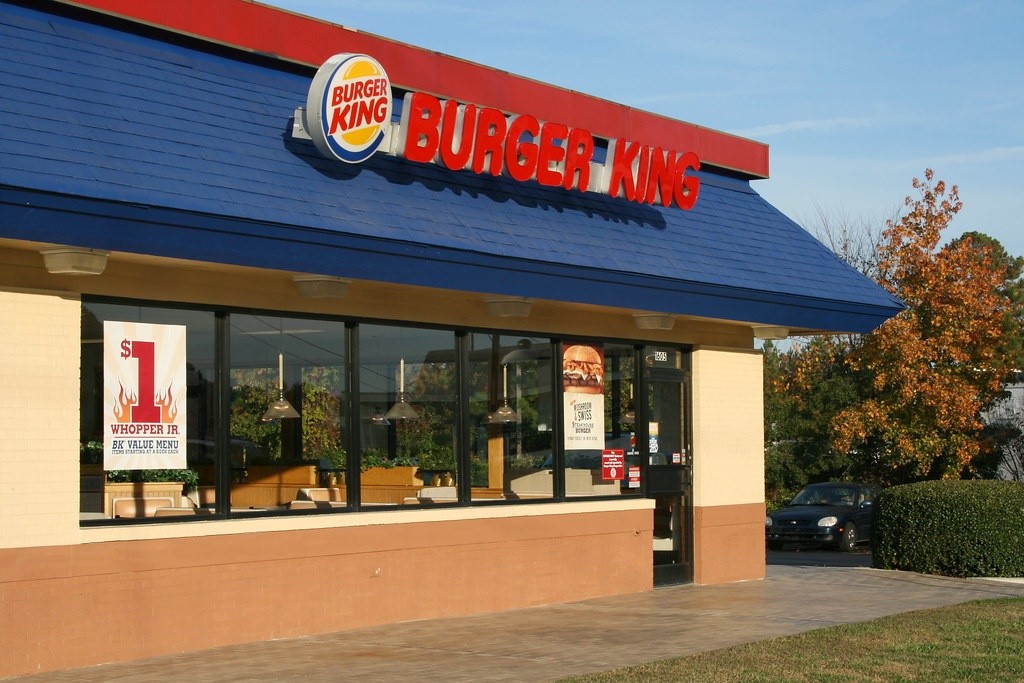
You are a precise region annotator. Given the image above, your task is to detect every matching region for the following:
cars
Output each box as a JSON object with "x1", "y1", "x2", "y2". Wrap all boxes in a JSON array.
[{"x1": 765, "y1": 482, "x2": 884, "y2": 552}]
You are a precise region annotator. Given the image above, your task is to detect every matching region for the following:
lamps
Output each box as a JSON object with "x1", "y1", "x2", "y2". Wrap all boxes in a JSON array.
[
  {"x1": 384, "y1": 359, "x2": 420, "y2": 419},
  {"x1": 489, "y1": 365, "x2": 522, "y2": 422},
  {"x1": 260, "y1": 352, "x2": 301, "y2": 420},
  {"x1": 500, "y1": 339, "x2": 551, "y2": 363}
]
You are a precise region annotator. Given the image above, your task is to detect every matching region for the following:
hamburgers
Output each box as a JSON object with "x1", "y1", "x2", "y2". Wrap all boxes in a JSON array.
[{"x1": 562, "y1": 345, "x2": 602, "y2": 394}]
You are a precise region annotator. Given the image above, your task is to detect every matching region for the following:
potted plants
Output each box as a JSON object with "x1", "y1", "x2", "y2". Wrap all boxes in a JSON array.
[
  {"x1": 196, "y1": 453, "x2": 320, "y2": 485},
  {"x1": 341, "y1": 448, "x2": 424, "y2": 487},
  {"x1": 80, "y1": 442, "x2": 200, "y2": 516},
  {"x1": 325, "y1": 472, "x2": 337, "y2": 486}
]
